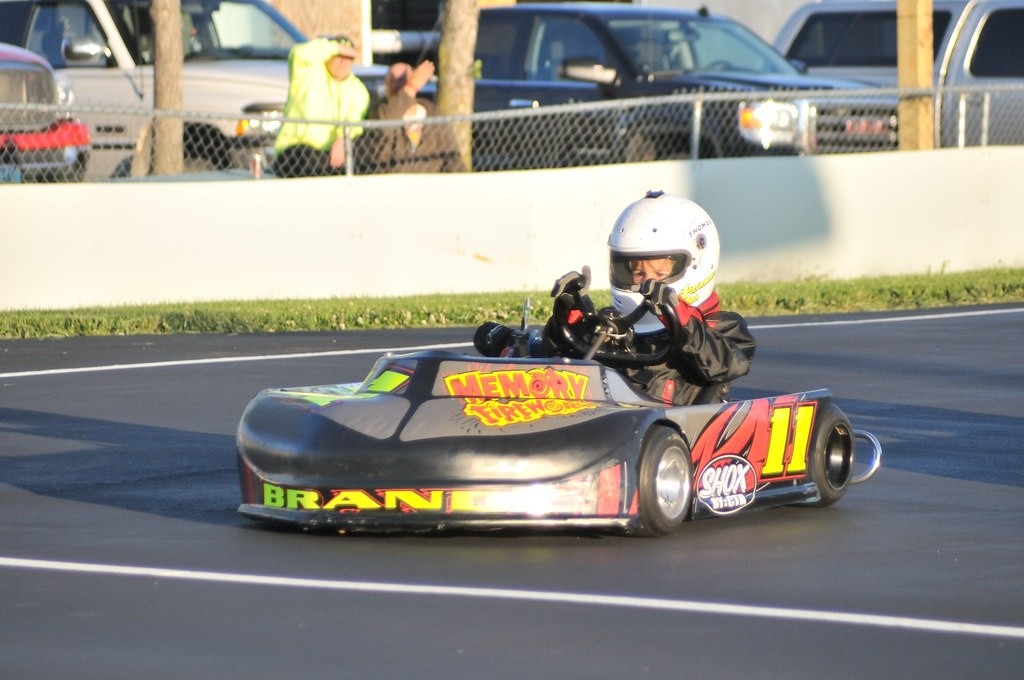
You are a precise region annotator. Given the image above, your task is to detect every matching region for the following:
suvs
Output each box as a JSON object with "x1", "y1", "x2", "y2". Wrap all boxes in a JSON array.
[
  {"x1": 0, "y1": 43, "x2": 92, "y2": 183},
  {"x1": 413, "y1": 6, "x2": 898, "y2": 174},
  {"x1": 768, "y1": 1, "x2": 1024, "y2": 154},
  {"x1": 23, "y1": 1, "x2": 391, "y2": 184}
]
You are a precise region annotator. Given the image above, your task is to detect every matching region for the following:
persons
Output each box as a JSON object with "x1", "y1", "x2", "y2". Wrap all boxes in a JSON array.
[
  {"x1": 272, "y1": 34, "x2": 372, "y2": 177},
  {"x1": 354, "y1": 60, "x2": 466, "y2": 174},
  {"x1": 473, "y1": 192, "x2": 757, "y2": 408}
]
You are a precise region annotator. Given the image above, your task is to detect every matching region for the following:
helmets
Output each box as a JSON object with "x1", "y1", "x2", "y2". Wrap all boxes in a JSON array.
[{"x1": 608, "y1": 192, "x2": 719, "y2": 334}]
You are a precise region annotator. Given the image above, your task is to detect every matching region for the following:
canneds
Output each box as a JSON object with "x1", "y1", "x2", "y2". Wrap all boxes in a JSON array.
[{"x1": 250, "y1": 153, "x2": 262, "y2": 178}]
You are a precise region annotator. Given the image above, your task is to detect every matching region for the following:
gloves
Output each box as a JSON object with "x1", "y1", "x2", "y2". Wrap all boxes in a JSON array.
[
  {"x1": 552, "y1": 267, "x2": 592, "y2": 300},
  {"x1": 630, "y1": 276, "x2": 680, "y2": 315}
]
